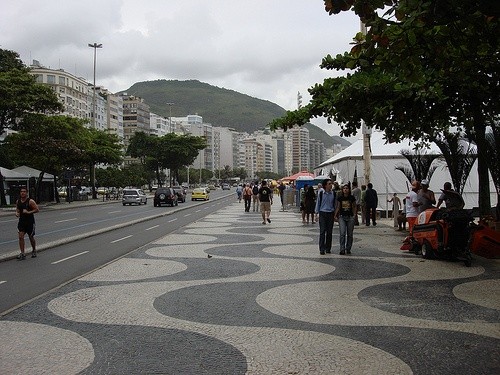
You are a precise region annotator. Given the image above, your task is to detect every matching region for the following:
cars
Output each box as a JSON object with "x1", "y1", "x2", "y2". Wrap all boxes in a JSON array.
[
  {"x1": 77, "y1": 186, "x2": 90, "y2": 195},
  {"x1": 153, "y1": 187, "x2": 179, "y2": 207},
  {"x1": 173, "y1": 189, "x2": 186, "y2": 203},
  {"x1": 191, "y1": 188, "x2": 209, "y2": 201},
  {"x1": 96, "y1": 187, "x2": 109, "y2": 194},
  {"x1": 209, "y1": 181, "x2": 237, "y2": 191},
  {"x1": 122, "y1": 189, "x2": 147, "y2": 205},
  {"x1": 172, "y1": 186, "x2": 187, "y2": 195},
  {"x1": 58, "y1": 186, "x2": 69, "y2": 197}
]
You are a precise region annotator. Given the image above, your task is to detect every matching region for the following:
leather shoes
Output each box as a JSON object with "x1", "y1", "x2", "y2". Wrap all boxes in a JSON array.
[{"x1": 319, "y1": 247, "x2": 331, "y2": 256}]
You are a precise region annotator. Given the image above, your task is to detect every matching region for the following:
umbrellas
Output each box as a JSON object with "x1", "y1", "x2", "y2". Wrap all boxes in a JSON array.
[{"x1": 285, "y1": 170, "x2": 316, "y2": 181}]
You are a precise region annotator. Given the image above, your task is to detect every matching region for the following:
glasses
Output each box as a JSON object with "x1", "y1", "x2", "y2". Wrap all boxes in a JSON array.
[{"x1": 326, "y1": 183, "x2": 332, "y2": 185}]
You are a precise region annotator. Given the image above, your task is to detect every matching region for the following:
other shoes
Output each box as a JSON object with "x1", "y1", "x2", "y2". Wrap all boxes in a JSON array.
[
  {"x1": 339, "y1": 249, "x2": 352, "y2": 255},
  {"x1": 236, "y1": 197, "x2": 407, "y2": 232}
]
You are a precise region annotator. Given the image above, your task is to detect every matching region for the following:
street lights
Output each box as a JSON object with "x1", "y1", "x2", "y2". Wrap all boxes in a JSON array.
[{"x1": 88, "y1": 42, "x2": 103, "y2": 187}]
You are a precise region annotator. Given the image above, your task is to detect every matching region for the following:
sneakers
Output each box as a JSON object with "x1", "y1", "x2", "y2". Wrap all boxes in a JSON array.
[{"x1": 16, "y1": 249, "x2": 36, "y2": 259}]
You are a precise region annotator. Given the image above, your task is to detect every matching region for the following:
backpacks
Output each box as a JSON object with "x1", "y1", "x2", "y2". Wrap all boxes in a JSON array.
[{"x1": 252, "y1": 185, "x2": 258, "y2": 195}]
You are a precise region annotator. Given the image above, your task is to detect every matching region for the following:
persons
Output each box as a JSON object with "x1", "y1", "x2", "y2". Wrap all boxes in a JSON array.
[
  {"x1": 359, "y1": 185, "x2": 368, "y2": 225},
  {"x1": 394, "y1": 198, "x2": 408, "y2": 232},
  {"x1": 416, "y1": 179, "x2": 437, "y2": 211},
  {"x1": 364, "y1": 182, "x2": 378, "y2": 226},
  {"x1": 350, "y1": 181, "x2": 362, "y2": 226},
  {"x1": 387, "y1": 192, "x2": 402, "y2": 220},
  {"x1": 300, "y1": 180, "x2": 351, "y2": 226},
  {"x1": 436, "y1": 182, "x2": 466, "y2": 209},
  {"x1": 258, "y1": 180, "x2": 273, "y2": 225},
  {"x1": 13, "y1": 188, "x2": 40, "y2": 261},
  {"x1": 250, "y1": 181, "x2": 260, "y2": 212},
  {"x1": 405, "y1": 180, "x2": 423, "y2": 235},
  {"x1": 237, "y1": 179, "x2": 296, "y2": 205},
  {"x1": 314, "y1": 179, "x2": 339, "y2": 255},
  {"x1": 243, "y1": 181, "x2": 252, "y2": 213},
  {"x1": 64, "y1": 184, "x2": 124, "y2": 203},
  {"x1": 334, "y1": 184, "x2": 358, "y2": 256}
]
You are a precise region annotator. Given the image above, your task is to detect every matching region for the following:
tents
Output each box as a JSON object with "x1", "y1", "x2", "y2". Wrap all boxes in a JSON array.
[
  {"x1": 311, "y1": 128, "x2": 500, "y2": 215},
  {"x1": 8, "y1": 165, "x2": 59, "y2": 204},
  {"x1": 0, "y1": 165, "x2": 31, "y2": 208}
]
